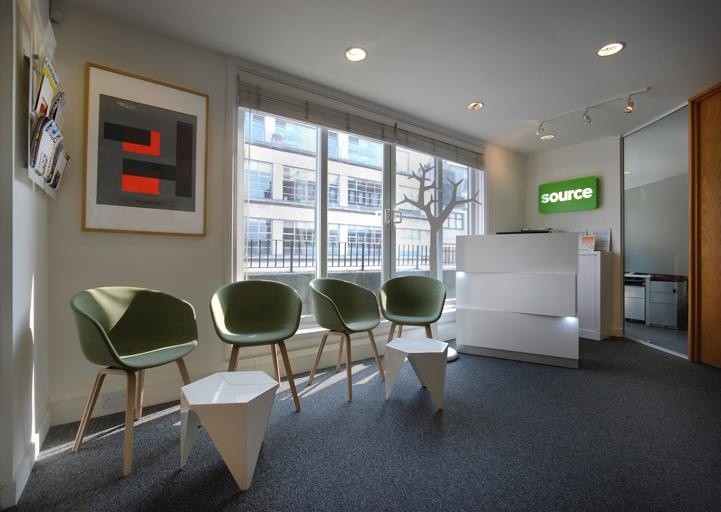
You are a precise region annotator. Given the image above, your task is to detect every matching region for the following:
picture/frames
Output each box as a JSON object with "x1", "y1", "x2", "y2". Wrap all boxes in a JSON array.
[
  {"x1": 81, "y1": 61, "x2": 208, "y2": 238},
  {"x1": 568, "y1": 226, "x2": 611, "y2": 252}
]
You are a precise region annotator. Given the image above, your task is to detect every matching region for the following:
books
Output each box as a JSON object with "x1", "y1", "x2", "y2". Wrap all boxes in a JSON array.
[{"x1": 29, "y1": 53, "x2": 72, "y2": 188}]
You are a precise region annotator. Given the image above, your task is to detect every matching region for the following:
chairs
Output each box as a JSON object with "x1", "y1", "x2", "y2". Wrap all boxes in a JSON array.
[
  {"x1": 208, "y1": 278, "x2": 304, "y2": 414},
  {"x1": 307, "y1": 276, "x2": 385, "y2": 404},
  {"x1": 69, "y1": 283, "x2": 201, "y2": 478},
  {"x1": 377, "y1": 274, "x2": 446, "y2": 343}
]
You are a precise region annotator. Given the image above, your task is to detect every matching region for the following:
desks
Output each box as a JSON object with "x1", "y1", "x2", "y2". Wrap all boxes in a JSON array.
[
  {"x1": 178, "y1": 370, "x2": 281, "y2": 491},
  {"x1": 383, "y1": 336, "x2": 450, "y2": 410}
]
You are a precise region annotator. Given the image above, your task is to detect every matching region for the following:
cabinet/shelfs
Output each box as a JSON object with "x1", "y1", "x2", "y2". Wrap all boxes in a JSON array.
[{"x1": 624, "y1": 272, "x2": 687, "y2": 330}]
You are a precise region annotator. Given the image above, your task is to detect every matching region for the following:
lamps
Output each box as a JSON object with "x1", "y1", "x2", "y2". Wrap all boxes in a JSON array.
[{"x1": 535, "y1": 85, "x2": 649, "y2": 141}]
